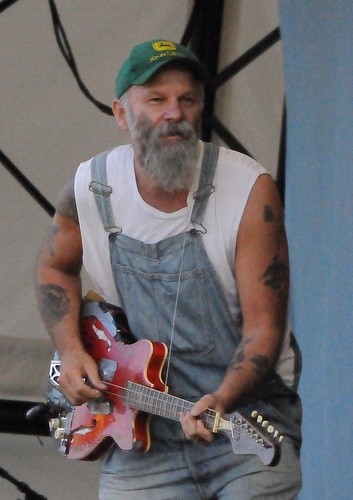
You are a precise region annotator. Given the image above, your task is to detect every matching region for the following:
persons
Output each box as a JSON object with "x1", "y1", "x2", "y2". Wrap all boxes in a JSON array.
[{"x1": 34, "y1": 39, "x2": 304, "y2": 498}]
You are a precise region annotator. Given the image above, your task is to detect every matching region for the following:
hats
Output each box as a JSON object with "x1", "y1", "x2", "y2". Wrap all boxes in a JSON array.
[{"x1": 116, "y1": 38, "x2": 206, "y2": 100}]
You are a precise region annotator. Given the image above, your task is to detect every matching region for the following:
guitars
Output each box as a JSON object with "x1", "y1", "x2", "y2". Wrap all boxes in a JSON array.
[{"x1": 26, "y1": 291, "x2": 283, "y2": 467}]
